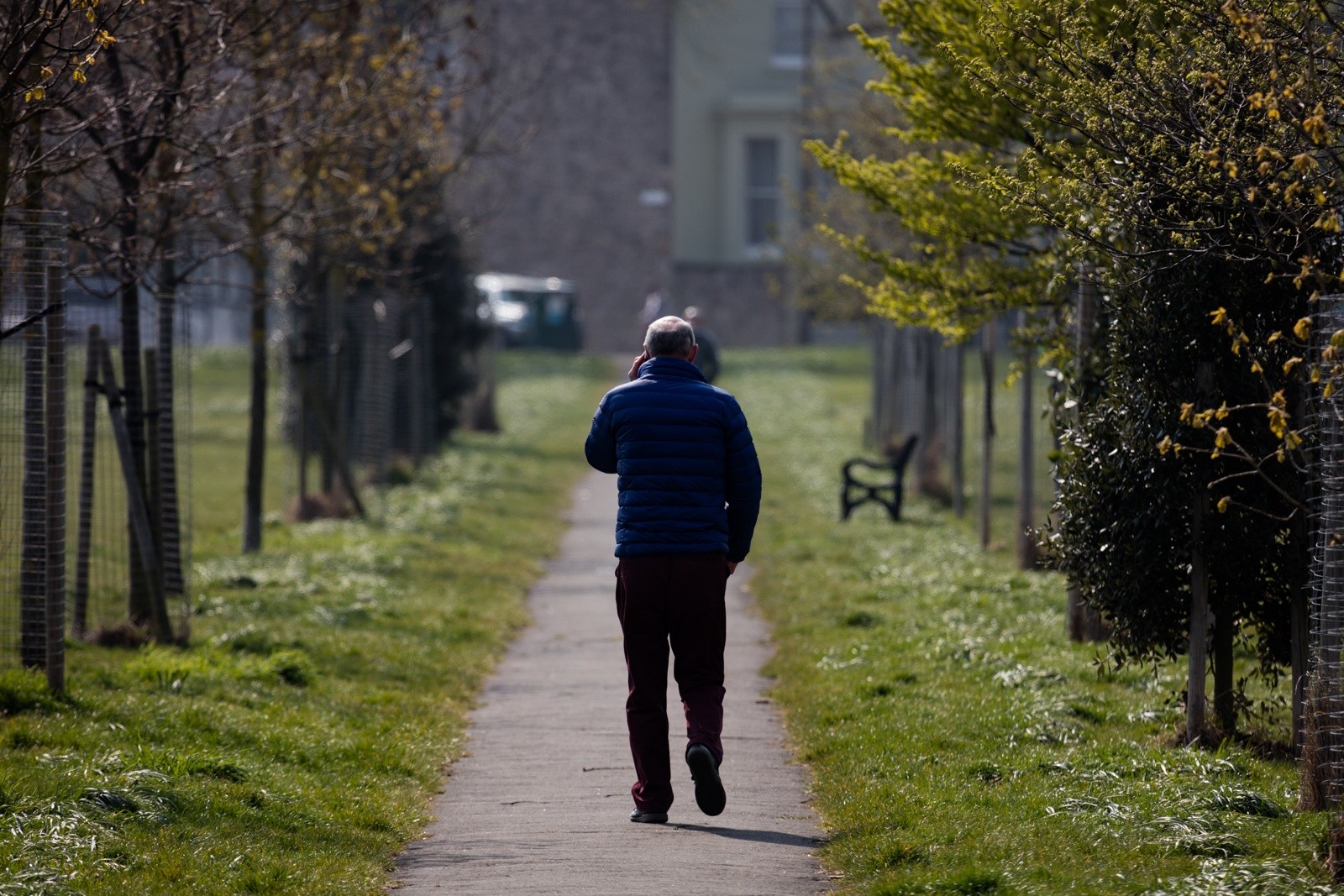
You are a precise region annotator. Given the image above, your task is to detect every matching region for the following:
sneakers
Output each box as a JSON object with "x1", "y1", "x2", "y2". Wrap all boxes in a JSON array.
[
  {"x1": 630, "y1": 807, "x2": 668, "y2": 823},
  {"x1": 687, "y1": 745, "x2": 726, "y2": 816}
]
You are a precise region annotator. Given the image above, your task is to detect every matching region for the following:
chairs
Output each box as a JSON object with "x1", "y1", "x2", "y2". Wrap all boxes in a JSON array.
[{"x1": 840, "y1": 435, "x2": 922, "y2": 518}]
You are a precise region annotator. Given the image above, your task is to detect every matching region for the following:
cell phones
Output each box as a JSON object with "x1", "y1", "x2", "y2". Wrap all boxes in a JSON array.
[{"x1": 644, "y1": 347, "x2": 651, "y2": 361}]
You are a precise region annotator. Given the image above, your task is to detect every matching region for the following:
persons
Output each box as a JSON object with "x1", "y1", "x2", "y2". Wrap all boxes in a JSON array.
[
  {"x1": 582, "y1": 315, "x2": 764, "y2": 824},
  {"x1": 682, "y1": 304, "x2": 719, "y2": 383}
]
